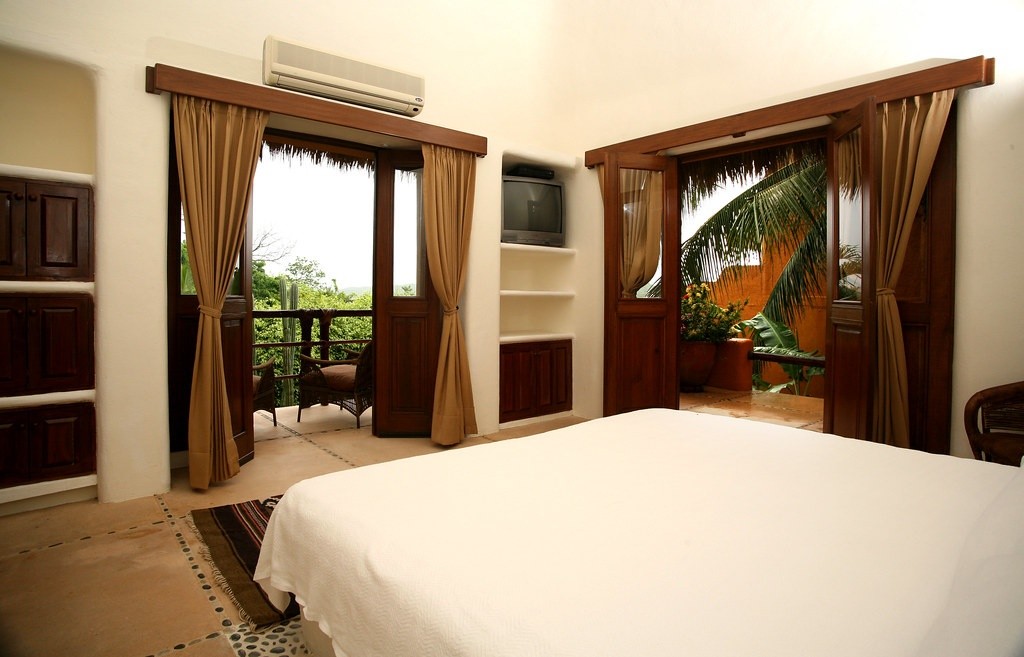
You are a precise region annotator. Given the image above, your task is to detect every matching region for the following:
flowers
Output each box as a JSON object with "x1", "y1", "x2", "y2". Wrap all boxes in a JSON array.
[{"x1": 680, "y1": 281, "x2": 750, "y2": 340}]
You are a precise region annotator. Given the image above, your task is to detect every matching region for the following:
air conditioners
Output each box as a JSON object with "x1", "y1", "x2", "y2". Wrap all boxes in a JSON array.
[{"x1": 264, "y1": 34, "x2": 425, "y2": 117}]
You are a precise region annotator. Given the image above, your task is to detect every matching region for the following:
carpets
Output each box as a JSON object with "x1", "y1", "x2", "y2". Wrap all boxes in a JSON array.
[{"x1": 185, "y1": 493, "x2": 302, "y2": 632}]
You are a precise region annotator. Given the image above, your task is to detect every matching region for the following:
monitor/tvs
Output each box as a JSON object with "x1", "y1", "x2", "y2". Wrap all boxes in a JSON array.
[{"x1": 501, "y1": 175, "x2": 566, "y2": 247}]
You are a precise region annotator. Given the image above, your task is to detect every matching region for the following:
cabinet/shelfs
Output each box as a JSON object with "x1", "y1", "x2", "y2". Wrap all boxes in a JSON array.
[
  {"x1": 0, "y1": 162, "x2": 102, "y2": 518},
  {"x1": 499, "y1": 245, "x2": 574, "y2": 420}
]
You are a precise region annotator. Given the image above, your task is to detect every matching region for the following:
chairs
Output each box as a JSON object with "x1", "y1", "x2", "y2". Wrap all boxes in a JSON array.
[
  {"x1": 252, "y1": 357, "x2": 277, "y2": 427},
  {"x1": 297, "y1": 341, "x2": 373, "y2": 428},
  {"x1": 964, "y1": 380, "x2": 1024, "y2": 467}
]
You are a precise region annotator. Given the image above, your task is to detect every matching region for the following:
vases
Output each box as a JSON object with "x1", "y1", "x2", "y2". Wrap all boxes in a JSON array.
[{"x1": 677, "y1": 338, "x2": 716, "y2": 392}]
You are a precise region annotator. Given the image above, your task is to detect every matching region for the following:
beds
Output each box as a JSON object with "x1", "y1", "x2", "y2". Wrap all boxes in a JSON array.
[{"x1": 252, "y1": 406, "x2": 1024, "y2": 657}]
